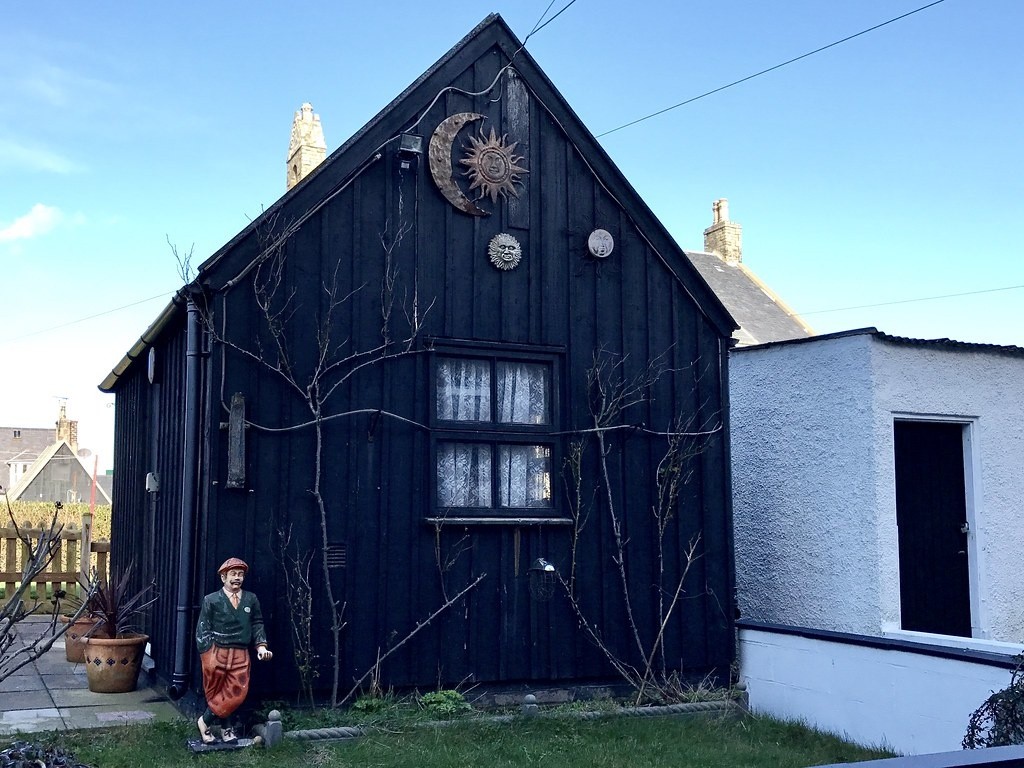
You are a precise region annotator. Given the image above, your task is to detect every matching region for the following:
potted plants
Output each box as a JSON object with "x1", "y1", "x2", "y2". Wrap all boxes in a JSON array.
[
  {"x1": 61, "y1": 571, "x2": 108, "y2": 662},
  {"x1": 74, "y1": 560, "x2": 160, "y2": 694}
]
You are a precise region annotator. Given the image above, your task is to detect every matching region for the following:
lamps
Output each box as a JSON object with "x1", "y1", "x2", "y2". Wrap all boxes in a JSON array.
[{"x1": 394, "y1": 132, "x2": 425, "y2": 172}]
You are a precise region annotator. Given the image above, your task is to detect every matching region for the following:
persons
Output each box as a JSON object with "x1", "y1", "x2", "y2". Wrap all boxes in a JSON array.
[{"x1": 196, "y1": 558, "x2": 273, "y2": 745}]
